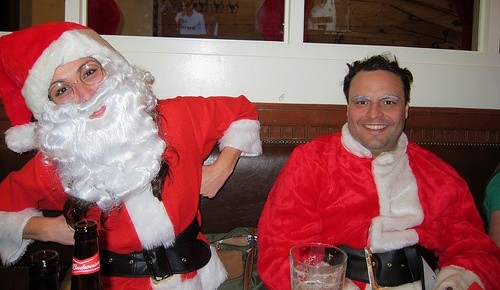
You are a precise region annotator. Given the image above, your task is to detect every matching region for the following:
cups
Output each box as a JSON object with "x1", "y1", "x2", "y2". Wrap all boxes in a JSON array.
[{"x1": 289, "y1": 242, "x2": 348, "y2": 290}]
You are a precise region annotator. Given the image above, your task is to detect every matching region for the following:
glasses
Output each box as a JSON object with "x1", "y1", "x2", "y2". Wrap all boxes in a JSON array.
[{"x1": 50, "y1": 64, "x2": 105, "y2": 101}]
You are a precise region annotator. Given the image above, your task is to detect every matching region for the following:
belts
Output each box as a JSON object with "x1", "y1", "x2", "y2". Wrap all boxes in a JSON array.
[
  {"x1": 325, "y1": 245, "x2": 438, "y2": 290},
  {"x1": 100, "y1": 217, "x2": 212, "y2": 284}
]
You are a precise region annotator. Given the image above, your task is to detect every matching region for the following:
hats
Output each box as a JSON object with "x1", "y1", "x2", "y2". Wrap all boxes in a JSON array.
[{"x1": 0, "y1": 22, "x2": 114, "y2": 152}]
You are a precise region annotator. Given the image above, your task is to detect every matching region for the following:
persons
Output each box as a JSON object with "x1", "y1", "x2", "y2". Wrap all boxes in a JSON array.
[
  {"x1": 256, "y1": 55, "x2": 500, "y2": 290},
  {"x1": 0, "y1": 21, "x2": 262, "y2": 290},
  {"x1": 483, "y1": 165, "x2": 500, "y2": 246}
]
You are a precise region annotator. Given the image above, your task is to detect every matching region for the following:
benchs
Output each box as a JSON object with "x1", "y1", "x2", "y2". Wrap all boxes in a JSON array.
[{"x1": 0, "y1": 139, "x2": 500, "y2": 270}]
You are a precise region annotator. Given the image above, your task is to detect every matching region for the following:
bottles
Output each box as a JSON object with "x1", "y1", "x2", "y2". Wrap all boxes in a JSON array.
[
  {"x1": 70, "y1": 220, "x2": 104, "y2": 290},
  {"x1": 31, "y1": 251, "x2": 58, "y2": 290}
]
